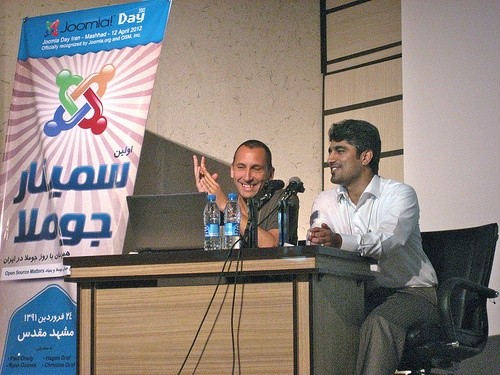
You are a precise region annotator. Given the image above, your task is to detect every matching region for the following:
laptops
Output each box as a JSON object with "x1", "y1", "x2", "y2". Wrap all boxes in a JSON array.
[{"x1": 125, "y1": 192, "x2": 209, "y2": 252}]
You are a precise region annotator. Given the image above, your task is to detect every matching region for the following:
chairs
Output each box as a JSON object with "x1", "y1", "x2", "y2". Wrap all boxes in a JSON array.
[{"x1": 405, "y1": 223, "x2": 498, "y2": 374}]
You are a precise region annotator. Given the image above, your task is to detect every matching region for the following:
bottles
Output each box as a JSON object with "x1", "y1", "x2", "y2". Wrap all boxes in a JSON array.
[
  {"x1": 223, "y1": 191, "x2": 242, "y2": 249},
  {"x1": 202, "y1": 194, "x2": 222, "y2": 249}
]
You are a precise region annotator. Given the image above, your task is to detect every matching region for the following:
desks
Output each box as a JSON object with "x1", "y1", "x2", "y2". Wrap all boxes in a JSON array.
[{"x1": 61, "y1": 245, "x2": 379, "y2": 375}]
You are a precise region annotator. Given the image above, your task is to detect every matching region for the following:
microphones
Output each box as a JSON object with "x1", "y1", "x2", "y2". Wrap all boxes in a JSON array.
[
  {"x1": 261, "y1": 179, "x2": 285, "y2": 194},
  {"x1": 278, "y1": 176, "x2": 301, "y2": 206}
]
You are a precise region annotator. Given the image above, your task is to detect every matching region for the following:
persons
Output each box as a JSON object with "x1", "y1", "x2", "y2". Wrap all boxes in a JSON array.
[
  {"x1": 306, "y1": 119, "x2": 439, "y2": 375},
  {"x1": 193, "y1": 140, "x2": 299, "y2": 247}
]
